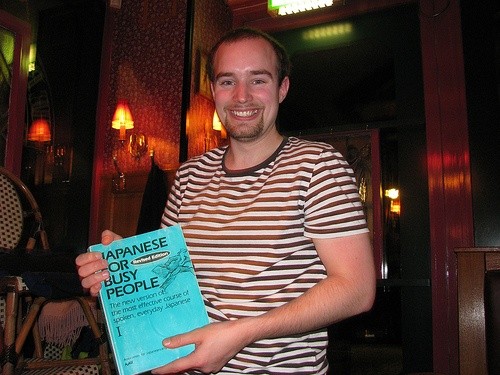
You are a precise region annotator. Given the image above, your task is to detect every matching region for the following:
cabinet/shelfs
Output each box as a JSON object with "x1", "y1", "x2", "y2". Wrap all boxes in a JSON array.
[{"x1": 455, "y1": 247, "x2": 500, "y2": 375}]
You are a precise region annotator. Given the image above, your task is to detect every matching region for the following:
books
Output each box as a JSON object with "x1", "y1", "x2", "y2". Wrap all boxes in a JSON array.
[{"x1": 87, "y1": 225, "x2": 211, "y2": 375}]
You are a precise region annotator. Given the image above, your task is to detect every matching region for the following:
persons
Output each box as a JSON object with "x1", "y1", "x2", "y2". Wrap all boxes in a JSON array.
[{"x1": 76, "y1": 28, "x2": 377, "y2": 375}]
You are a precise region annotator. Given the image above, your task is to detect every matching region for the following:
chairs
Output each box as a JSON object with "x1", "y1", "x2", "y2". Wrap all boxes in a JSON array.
[{"x1": 1, "y1": 166, "x2": 113, "y2": 375}]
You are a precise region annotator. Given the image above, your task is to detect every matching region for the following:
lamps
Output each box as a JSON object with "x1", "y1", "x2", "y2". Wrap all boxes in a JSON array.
[
  {"x1": 112, "y1": 103, "x2": 134, "y2": 140},
  {"x1": 28, "y1": 118, "x2": 51, "y2": 154}
]
